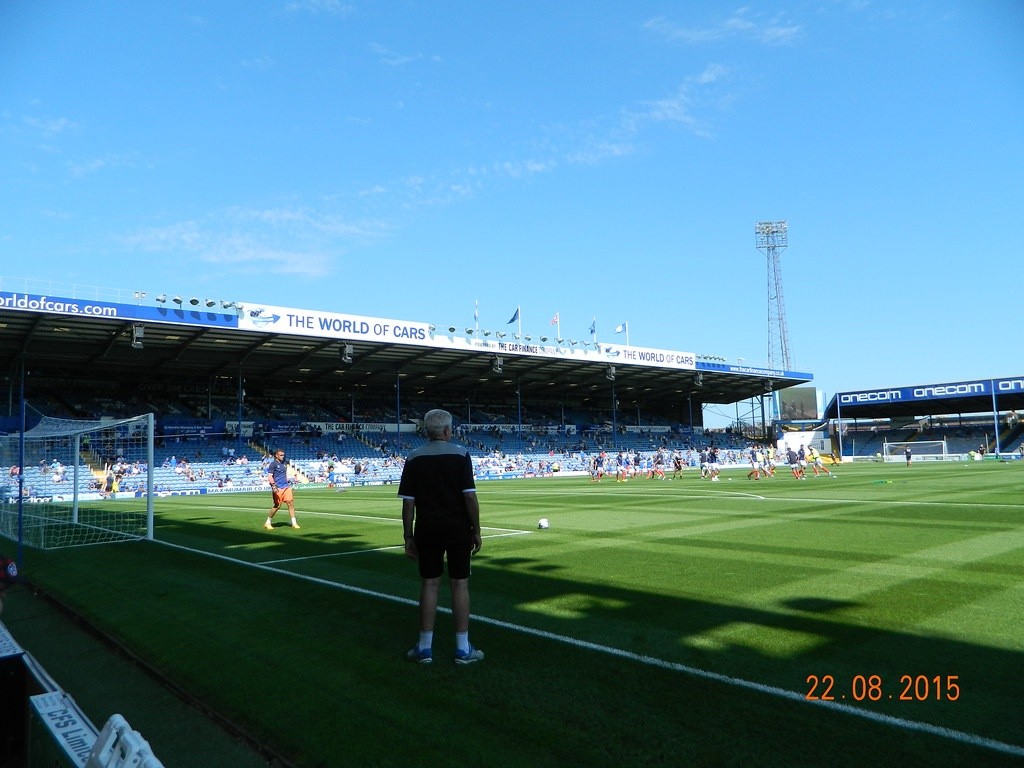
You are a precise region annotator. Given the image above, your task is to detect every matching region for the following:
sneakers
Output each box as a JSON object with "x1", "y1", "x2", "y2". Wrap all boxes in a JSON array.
[
  {"x1": 454, "y1": 642, "x2": 484, "y2": 664},
  {"x1": 406, "y1": 642, "x2": 433, "y2": 663},
  {"x1": 291, "y1": 522, "x2": 300, "y2": 528},
  {"x1": 264, "y1": 522, "x2": 274, "y2": 529}
]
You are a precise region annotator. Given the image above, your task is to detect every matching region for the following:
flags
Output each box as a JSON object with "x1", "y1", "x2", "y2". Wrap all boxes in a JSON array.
[
  {"x1": 549, "y1": 313, "x2": 558, "y2": 327},
  {"x1": 588, "y1": 319, "x2": 596, "y2": 335},
  {"x1": 474, "y1": 301, "x2": 479, "y2": 321},
  {"x1": 506, "y1": 307, "x2": 519, "y2": 325},
  {"x1": 613, "y1": 323, "x2": 626, "y2": 334}
]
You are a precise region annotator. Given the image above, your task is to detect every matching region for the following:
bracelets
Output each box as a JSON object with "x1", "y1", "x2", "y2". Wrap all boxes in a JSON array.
[
  {"x1": 472, "y1": 526, "x2": 481, "y2": 534},
  {"x1": 270, "y1": 482, "x2": 276, "y2": 489}
]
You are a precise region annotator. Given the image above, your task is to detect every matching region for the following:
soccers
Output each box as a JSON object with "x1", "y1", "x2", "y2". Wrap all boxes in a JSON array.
[{"x1": 539, "y1": 518, "x2": 550, "y2": 529}]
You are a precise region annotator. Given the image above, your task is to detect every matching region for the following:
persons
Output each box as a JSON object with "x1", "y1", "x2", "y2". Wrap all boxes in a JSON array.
[
  {"x1": 8, "y1": 380, "x2": 843, "y2": 483},
  {"x1": 873, "y1": 409, "x2": 1024, "y2": 463},
  {"x1": 265, "y1": 449, "x2": 302, "y2": 530},
  {"x1": 398, "y1": 408, "x2": 487, "y2": 666}
]
